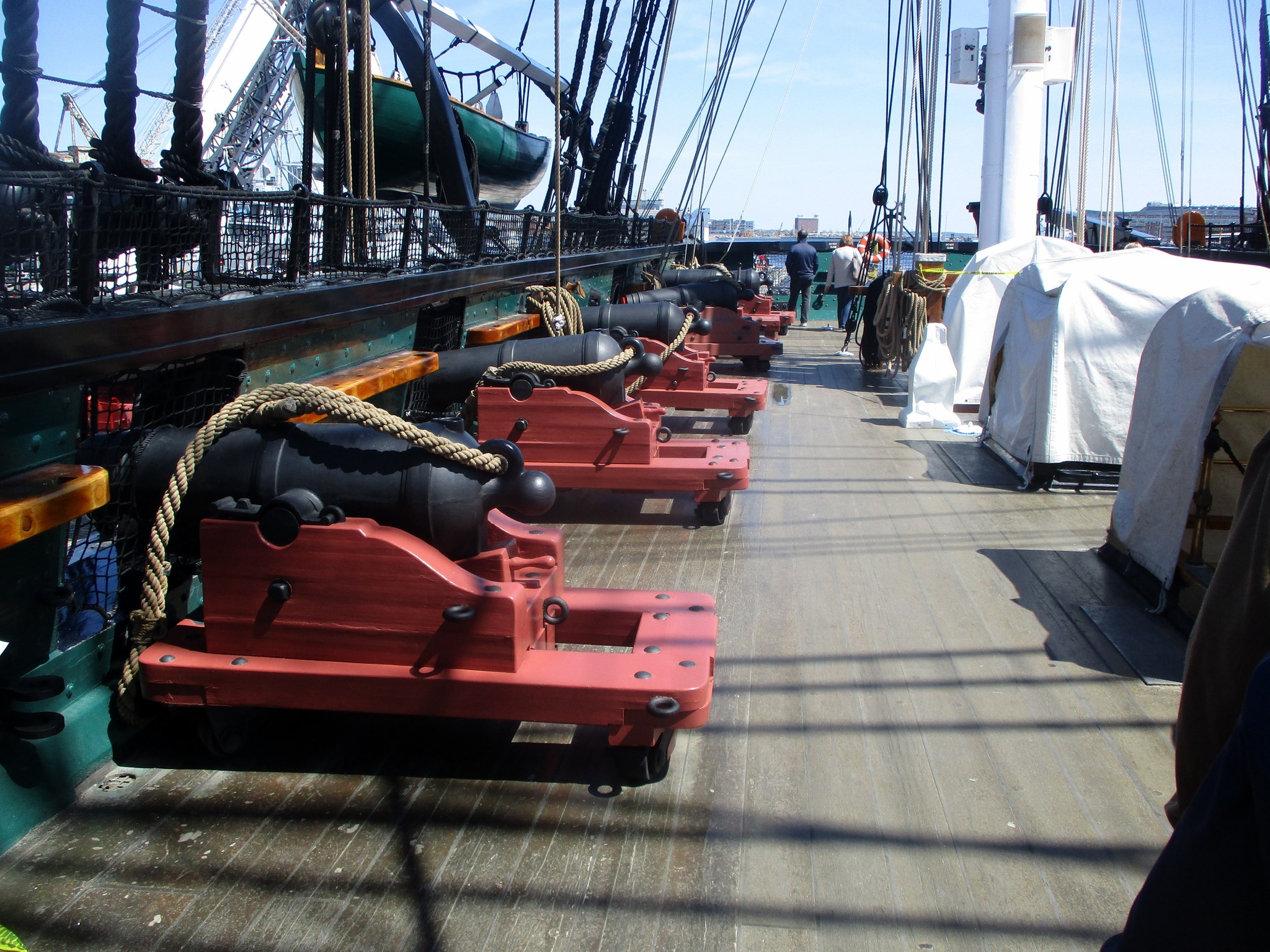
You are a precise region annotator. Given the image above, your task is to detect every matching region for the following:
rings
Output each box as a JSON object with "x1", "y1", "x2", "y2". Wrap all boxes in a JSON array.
[{"x1": 824, "y1": 289, "x2": 825, "y2": 290}]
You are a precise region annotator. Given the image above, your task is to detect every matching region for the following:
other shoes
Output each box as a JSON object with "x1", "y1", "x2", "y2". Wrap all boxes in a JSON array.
[
  {"x1": 800, "y1": 322, "x2": 806, "y2": 327},
  {"x1": 839, "y1": 327, "x2": 846, "y2": 332}
]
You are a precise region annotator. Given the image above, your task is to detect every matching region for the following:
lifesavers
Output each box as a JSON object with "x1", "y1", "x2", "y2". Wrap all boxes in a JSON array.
[{"x1": 857, "y1": 233, "x2": 890, "y2": 264}]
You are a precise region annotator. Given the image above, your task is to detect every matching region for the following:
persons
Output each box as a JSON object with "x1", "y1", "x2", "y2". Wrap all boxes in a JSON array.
[
  {"x1": 823, "y1": 234, "x2": 867, "y2": 333},
  {"x1": 759, "y1": 254, "x2": 770, "y2": 267},
  {"x1": 785, "y1": 229, "x2": 819, "y2": 326}
]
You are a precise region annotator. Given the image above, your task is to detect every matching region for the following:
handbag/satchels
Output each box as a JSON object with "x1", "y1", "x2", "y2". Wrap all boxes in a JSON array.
[{"x1": 852, "y1": 247, "x2": 865, "y2": 285}]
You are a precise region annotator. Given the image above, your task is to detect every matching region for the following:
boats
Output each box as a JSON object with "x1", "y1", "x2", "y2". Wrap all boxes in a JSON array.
[{"x1": 288, "y1": 50, "x2": 550, "y2": 214}]
